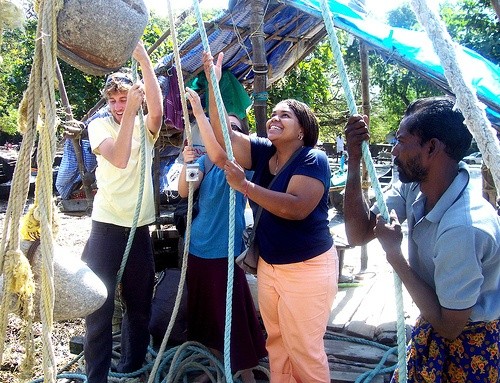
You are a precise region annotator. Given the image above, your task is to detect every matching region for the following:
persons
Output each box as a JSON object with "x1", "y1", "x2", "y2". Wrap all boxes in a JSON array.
[
  {"x1": 80, "y1": 38, "x2": 166, "y2": 383},
  {"x1": 177, "y1": 86, "x2": 268, "y2": 383},
  {"x1": 335, "y1": 132, "x2": 344, "y2": 163},
  {"x1": 200, "y1": 50, "x2": 340, "y2": 383},
  {"x1": 342, "y1": 94, "x2": 500, "y2": 383}
]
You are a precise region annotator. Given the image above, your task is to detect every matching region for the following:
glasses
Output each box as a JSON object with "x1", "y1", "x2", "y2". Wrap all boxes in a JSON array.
[{"x1": 231, "y1": 125, "x2": 246, "y2": 134}]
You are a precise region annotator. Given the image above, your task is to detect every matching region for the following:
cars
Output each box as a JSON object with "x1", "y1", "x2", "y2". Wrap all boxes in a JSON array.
[{"x1": 464, "y1": 152, "x2": 482, "y2": 165}]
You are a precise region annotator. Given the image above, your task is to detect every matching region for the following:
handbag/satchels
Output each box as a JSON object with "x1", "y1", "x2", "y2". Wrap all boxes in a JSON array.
[
  {"x1": 173, "y1": 188, "x2": 200, "y2": 236},
  {"x1": 235, "y1": 226, "x2": 260, "y2": 276}
]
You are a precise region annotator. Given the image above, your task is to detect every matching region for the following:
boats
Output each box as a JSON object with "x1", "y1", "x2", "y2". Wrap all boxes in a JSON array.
[{"x1": 330, "y1": 152, "x2": 396, "y2": 217}]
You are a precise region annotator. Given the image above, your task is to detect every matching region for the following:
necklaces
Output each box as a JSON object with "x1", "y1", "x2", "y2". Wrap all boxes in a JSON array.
[{"x1": 273, "y1": 155, "x2": 280, "y2": 176}]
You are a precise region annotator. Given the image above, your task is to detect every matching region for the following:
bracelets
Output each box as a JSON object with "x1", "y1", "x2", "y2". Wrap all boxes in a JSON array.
[{"x1": 243, "y1": 181, "x2": 250, "y2": 197}]
